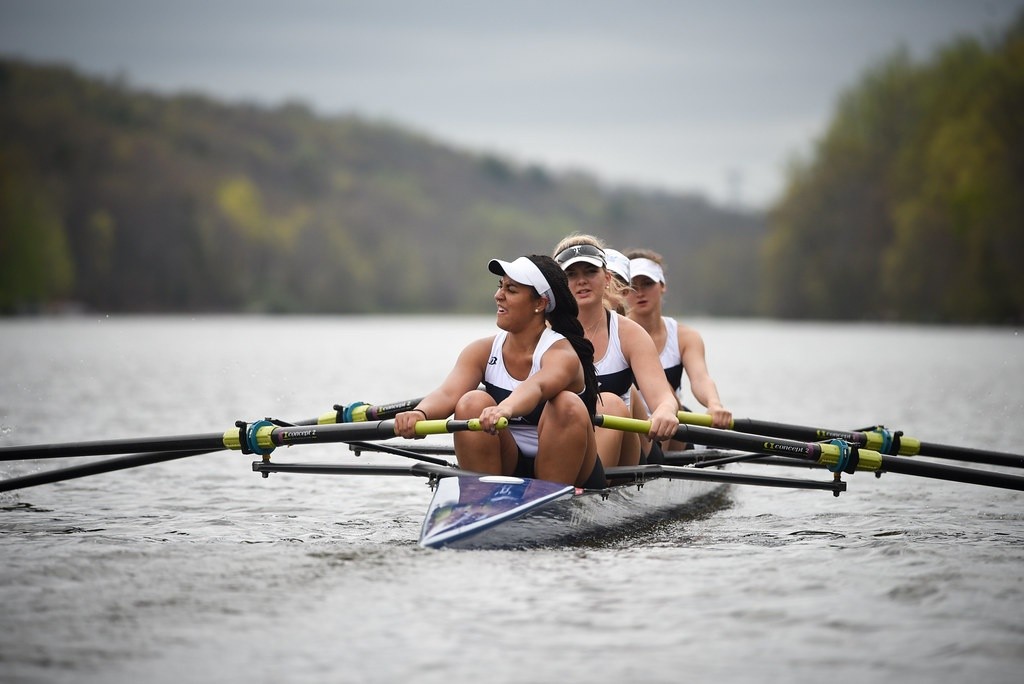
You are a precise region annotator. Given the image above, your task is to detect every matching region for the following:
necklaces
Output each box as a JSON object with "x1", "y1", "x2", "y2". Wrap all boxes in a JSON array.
[{"x1": 584, "y1": 308, "x2": 605, "y2": 342}]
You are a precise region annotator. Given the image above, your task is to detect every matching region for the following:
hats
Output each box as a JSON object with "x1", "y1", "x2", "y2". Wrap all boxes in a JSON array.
[
  {"x1": 601, "y1": 248, "x2": 631, "y2": 288},
  {"x1": 553, "y1": 243, "x2": 607, "y2": 272},
  {"x1": 485, "y1": 256, "x2": 556, "y2": 314},
  {"x1": 630, "y1": 257, "x2": 666, "y2": 286}
]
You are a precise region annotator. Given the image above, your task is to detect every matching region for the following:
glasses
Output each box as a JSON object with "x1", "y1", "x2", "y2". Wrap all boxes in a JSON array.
[{"x1": 557, "y1": 245, "x2": 607, "y2": 264}]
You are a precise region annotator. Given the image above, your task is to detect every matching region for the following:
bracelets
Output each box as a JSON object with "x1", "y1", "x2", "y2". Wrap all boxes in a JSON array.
[{"x1": 411, "y1": 409, "x2": 427, "y2": 420}]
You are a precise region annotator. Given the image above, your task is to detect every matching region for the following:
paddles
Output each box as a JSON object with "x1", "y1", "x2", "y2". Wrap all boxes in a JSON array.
[
  {"x1": 591, "y1": 408, "x2": 1024, "y2": 496},
  {"x1": 0, "y1": 384, "x2": 508, "y2": 495}
]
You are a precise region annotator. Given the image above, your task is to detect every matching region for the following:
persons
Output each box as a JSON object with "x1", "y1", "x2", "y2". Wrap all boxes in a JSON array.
[{"x1": 394, "y1": 236, "x2": 732, "y2": 486}]
